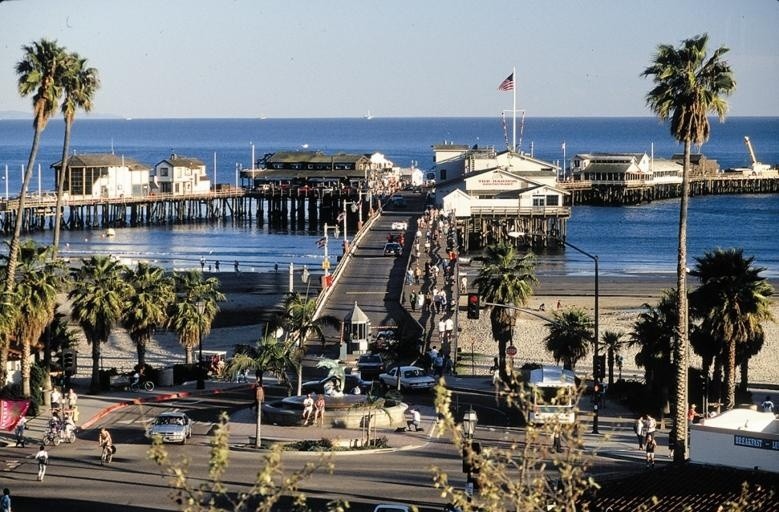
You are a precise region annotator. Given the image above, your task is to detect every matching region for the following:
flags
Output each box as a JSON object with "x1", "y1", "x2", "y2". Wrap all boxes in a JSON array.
[
  {"x1": 498, "y1": 73, "x2": 515, "y2": 92},
  {"x1": 0, "y1": 400, "x2": 30, "y2": 432}
]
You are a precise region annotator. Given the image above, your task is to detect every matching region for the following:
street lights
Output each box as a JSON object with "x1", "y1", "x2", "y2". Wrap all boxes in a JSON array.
[
  {"x1": 460, "y1": 403, "x2": 482, "y2": 511},
  {"x1": 322, "y1": 222, "x2": 340, "y2": 289},
  {"x1": 287, "y1": 261, "x2": 310, "y2": 337},
  {"x1": 505, "y1": 297, "x2": 516, "y2": 376},
  {"x1": 684, "y1": 265, "x2": 710, "y2": 420},
  {"x1": 341, "y1": 185, "x2": 384, "y2": 257},
  {"x1": 194, "y1": 297, "x2": 208, "y2": 390},
  {"x1": 507, "y1": 230, "x2": 604, "y2": 398}
]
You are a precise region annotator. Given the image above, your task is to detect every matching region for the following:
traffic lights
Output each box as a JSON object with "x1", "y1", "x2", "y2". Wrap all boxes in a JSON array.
[
  {"x1": 594, "y1": 382, "x2": 603, "y2": 393},
  {"x1": 592, "y1": 354, "x2": 606, "y2": 379},
  {"x1": 467, "y1": 292, "x2": 480, "y2": 320},
  {"x1": 62, "y1": 350, "x2": 77, "y2": 370}
]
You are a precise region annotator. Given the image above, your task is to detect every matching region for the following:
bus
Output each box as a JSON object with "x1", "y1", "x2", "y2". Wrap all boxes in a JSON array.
[{"x1": 528, "y1": 365, "x2": 578, "y2": 427}]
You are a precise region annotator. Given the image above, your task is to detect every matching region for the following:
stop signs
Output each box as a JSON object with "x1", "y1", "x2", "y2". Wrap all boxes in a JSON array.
[{"x1": 506, "y1": 345, "x2": 518, "y2": 357}]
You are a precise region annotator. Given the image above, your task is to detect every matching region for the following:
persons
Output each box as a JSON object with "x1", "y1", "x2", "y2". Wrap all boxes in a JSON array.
[
  {"x1": 68, "y1": 389, "x2": 78, "y2": 409},
  {"x1": 386, "y1": 233, "x2": 393, "y2": 243},
  {"x1": 689, "y1": 404, "x2": 700, "y2": 424},
  {"x1": 490, "y1": 356, "x2": 504, "y2": 385},
  {"x1": 131, "y1": 366, "x2": 146, "y2": 387},
  {"x1": 99, "y1": 427, "x2": 113, "y2": 454},
  {"x1": 462, "y1": 276, "x2": 468, "y2": 288},
  {"x1": 248, "y1": 381, "x2": 264, "y2": 412},
  {"x1": 645, "y1": 433, "x2": 657, "y2": 466},
  {"x1": 644, "y1": 414, "x2": 657, "y2": 437},
  {"x1": 598, "y1": 377, "x2": 608, "y2": 409},
  {"x1": 407, "y1": 408, "x2": 424, "y2": 432},
  {"x1": 209, "y1": 355, "x2": 216, "y2": 372},
  {"x1": 213, "y1": 353, "x2": 219, "y2": 364},
  {"x1": 51, "y1": 386, "x2": 62, "y2": 408},
  {"x1": 35, "y1": 446, "x2": 49, "y2": 481},
  {"x1": 312, "y1": 394, "x2": 326, "y2": 425},
  {"x1": 636, "y1": 416, "x2": 645, "y2": 450},
  {"x1": 406, "y1": 204, "x2": 456, "y2": 376},
  {"x1": 761, "y1": 396, "x2": 774, "y2": 412},
  {"x1": 0, "y1": 488, "x2": 11, "y2": 512},
  {"x1": 15, "y1": 414, "x2": 26, "y2": 436},
  {"x1": 398, "y1": 233, "x2": 405, "y2": 247},
  {"x1": 302, "y1": 393, "x2": 314, "y2": 426},
  {"x1": 667, "y1": 432, "x2": 674, "y2": 458}
]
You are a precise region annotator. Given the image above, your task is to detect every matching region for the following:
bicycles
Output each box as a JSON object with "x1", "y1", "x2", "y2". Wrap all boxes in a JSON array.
[
  {"x1": 98, "y1": 441, "x2": 115, "y2": 467},
  {"x1": 42, "y1": 419, "x2": 77, "y2": 445},
  {"x1": 130, "y1": 374, "x2": 158, "y2": 392}
]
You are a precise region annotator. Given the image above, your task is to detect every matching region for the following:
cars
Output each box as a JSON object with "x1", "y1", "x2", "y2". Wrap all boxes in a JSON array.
[
  {"x1": 299, "y1": 327, "x2": 437, "y2": 402},
  {"x1": 388, "y1": 183, "x2": 429, "y2": 208},
  {"x1": 374, "y1": 503, "x2": 413, "y2": 512},
  {"x1": 385, "y1": 219, "x2": 408, "y2": 258},
  {"x1": 149, "y1": 410, "x2": 195, "y2": 446}
]
